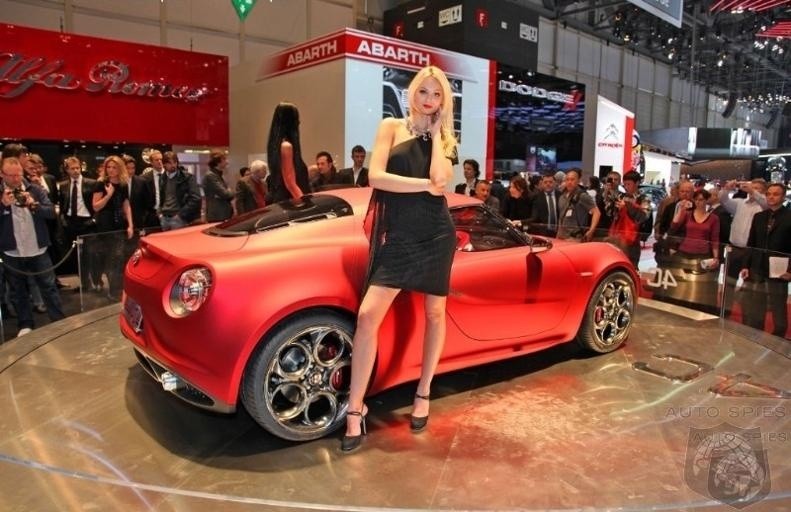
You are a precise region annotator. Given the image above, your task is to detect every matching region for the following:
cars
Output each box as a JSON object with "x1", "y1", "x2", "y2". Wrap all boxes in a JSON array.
[{"x1": 119, "y1": 104, "x2": 642, "y2": 443}]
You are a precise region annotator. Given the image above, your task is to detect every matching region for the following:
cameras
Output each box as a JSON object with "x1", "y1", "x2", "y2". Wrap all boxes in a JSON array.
[{"x1": 8, "y1": 187, "x2": 27, "y2": 205}]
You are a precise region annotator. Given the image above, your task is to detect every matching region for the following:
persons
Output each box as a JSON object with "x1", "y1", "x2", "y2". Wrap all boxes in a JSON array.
[
  {"x1": 338, "y1": 62, "x2": 459, "y2": 455},
  {"x1": 1, "y1": 140, "x2": 791, "y2": 341},
  {"x1": 268, "y1": 99, "x2": 314, "y2": 206}
]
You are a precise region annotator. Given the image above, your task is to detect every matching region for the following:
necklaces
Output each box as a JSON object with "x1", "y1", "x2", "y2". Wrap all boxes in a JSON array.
[{"x1": 404, "y1": 109, "x2": 437, "y2": 142}]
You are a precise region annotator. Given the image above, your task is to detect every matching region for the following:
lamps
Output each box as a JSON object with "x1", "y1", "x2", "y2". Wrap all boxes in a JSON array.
[{"x1": 610, "y1": 0, "x2": 791, "y2": 116}]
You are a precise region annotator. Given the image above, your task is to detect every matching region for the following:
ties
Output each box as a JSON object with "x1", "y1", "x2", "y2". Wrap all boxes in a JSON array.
[
  {"x1": 72, "y1": 181, "x2": 77, "y2": 216},
  {"x1": 548, "y1": 194, "x2": 555, "y2": 224}
]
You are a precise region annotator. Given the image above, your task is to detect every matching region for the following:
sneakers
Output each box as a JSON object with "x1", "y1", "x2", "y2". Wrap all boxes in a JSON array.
[
  {"x1": 36, "y1": 305, "x2": 47, "y2": 314},
  {"x1": 17, "y1": 328, "x2": 32, "y2": 337}
]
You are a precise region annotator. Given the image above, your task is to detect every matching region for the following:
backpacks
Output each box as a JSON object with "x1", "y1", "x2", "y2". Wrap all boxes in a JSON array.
[{"x1": 636, "y1": 194, "x2": 652, "y2": 242}]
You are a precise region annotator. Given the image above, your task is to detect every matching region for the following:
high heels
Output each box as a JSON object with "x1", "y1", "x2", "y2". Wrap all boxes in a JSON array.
[
  {"x1": 411, "y1": 393, "x2": 430, "y2": 431},
  {"x1": 342, "y1": 404, "x2": 368, "y2": 451}
]
